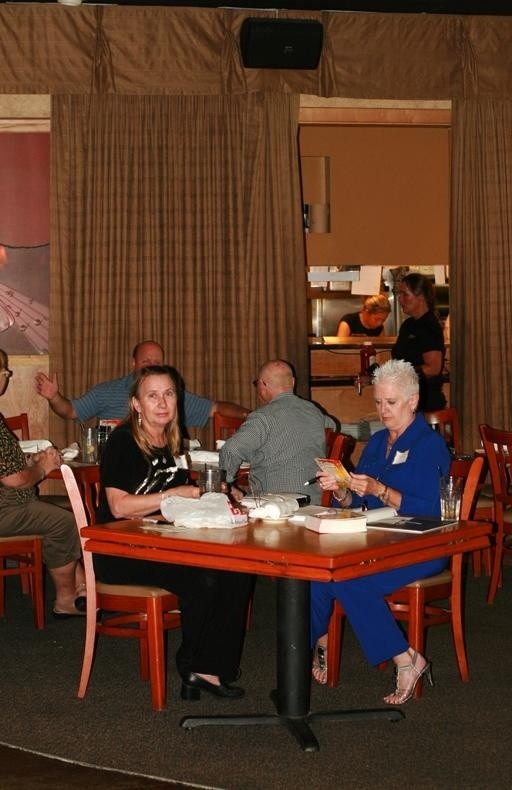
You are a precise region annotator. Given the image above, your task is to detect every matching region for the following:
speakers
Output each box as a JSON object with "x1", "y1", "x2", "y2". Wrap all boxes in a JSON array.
[{"x1": 240, "y1": 18, "x2": 324, "y2": 70}]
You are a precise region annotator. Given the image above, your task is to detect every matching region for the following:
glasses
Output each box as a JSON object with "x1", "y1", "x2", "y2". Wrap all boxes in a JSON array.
[{"x1": 252, "y1": 378, "x2": 267, "y2": 388}]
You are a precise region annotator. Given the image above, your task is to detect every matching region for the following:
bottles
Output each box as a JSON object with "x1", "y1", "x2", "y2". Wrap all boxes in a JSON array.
[{"x1": 360, "y1": 342, "x2": 376, "y2": 375}]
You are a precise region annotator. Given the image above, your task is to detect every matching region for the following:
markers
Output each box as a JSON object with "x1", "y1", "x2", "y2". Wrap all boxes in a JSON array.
[{"x1": 303, "y1": 476, "x2": 322, "y2": 487}]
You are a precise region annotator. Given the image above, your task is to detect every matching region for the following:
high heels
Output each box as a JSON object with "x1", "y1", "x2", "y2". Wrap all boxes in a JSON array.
[
  {"x1": 181, "y1": 669, "x2": 246, "y2": 704},
  {"x1": 311, "y1": 640, "x2": 331, "y2": 686},
  {"x1": 381, "y1": 649, "x2": 434, "y2": 704}
]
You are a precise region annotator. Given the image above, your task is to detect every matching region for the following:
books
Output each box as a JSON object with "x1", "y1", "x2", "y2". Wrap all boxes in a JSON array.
[{"x1": 366, "y1": 516, "x2": 457, "y2": 535}]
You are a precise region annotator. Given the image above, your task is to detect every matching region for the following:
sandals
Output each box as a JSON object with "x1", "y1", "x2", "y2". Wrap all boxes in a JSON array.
[
  {"x1": 55, "y1": 607, "x2": 102, "y2": 621},
  {"x1": 75, "y1": 587, "x2": 89, "y2": 608}
]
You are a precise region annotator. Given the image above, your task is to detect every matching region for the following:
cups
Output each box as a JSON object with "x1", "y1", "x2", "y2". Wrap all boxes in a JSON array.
[
  {"x1": 438, "y1": 475, "x2": 462, "y2": 522},
  {"x1": 219, "y1": 426, "x2": 234, "y2": 442},
  {"x1": 80, "y1": 426, "x2": 98, "y2": 464},
  {"x1": 198, "y1": 468, "x2": 222, "y2": 494}
]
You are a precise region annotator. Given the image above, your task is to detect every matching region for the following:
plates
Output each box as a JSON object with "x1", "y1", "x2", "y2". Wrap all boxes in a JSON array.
[{"x1": 250, "y1": 516, "x2": 292, "y2": 526}]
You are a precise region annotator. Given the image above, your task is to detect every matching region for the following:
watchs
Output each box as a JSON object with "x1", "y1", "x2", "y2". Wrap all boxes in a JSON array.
[{"x1": 331, "y1": 489, "x2": 350, "y2": 504}]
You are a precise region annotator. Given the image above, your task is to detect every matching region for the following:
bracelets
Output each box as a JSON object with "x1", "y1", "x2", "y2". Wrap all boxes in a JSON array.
[
  {"x1": 158, "y1": 489, "x2": 165, "y2": 502},
  {"x1": 378, "y1": 485, "x2": 389, "y2": 502}
]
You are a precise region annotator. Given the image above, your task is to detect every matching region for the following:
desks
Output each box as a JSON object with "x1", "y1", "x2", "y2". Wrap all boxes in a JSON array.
[
  {"x1": 45, "y1": 450, "x2": 251, "y2": 481},
  {"x1": 80, "y1": 516, "x2": 495, "y2": 753}
]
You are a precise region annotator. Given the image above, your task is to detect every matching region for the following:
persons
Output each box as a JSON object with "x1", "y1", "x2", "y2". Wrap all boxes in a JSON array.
[
  {"x1": 216, "y1": 361, "x2": 336, "y2": 507},
  {"x1": 312, "y1": 359, "x2": 450, "y2": 704},
  {"x1": 36, "y1": 340, "x2": 253, "y2": 429},
  {"x1": 336, "y1": 295, "x2": 392, "y2": 337},
  {"x1": 357, "y1": 273, "x2": 446, "y2": 413},
  {"x1": 94, "y1": 365, "x2": 256, "y2": 701},
  {"x1": 2, "y1": 351, "x2": 116, "y2": 619}
]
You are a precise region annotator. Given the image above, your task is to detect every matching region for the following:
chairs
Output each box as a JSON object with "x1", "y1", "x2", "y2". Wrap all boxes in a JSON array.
[
  {"x1": 246, "y1": 433, "x2": 358, "y2": 630},
  {"x1": 212, "y1": 408, "x2": 249, "y2": 450},
  {"x1": 2, "y1": 414, "x2": 29, "y2": 441},
  {"x1": 325, "y1": 452, "x2": 486, "y2": 699},
  {"x1": 60, "y1": 461, "x2": 184, "y2": 714},
  {"x1": 0, "y1": 534, "x2": 44, "y2": 629},
  {"x1": 480, "y1": 421, "x2": 512, "y2": 604}
]
